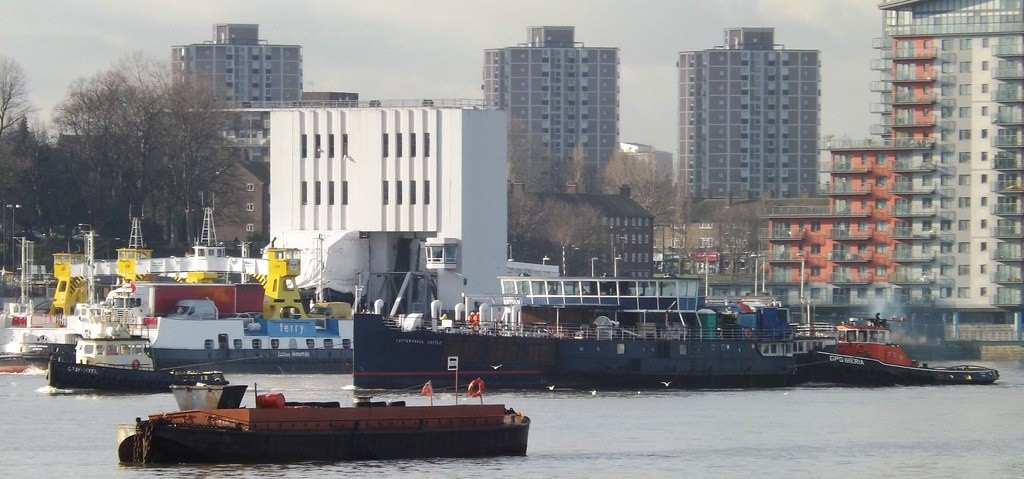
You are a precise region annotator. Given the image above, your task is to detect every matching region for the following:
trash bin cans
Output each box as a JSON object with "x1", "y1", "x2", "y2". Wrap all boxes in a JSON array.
[
  {"x1": 696, "y1": 308, "x2": 717, "y2": 341},
  {"x1": 763, "y1": 308, "x2": 784, "y2": 341},
  {"x1": 737, "y1": 311, "x2": 760, "y2": 341}
]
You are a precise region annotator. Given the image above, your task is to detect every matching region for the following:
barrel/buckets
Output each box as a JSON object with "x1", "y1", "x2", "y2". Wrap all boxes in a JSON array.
[{"x1": 257, "y1": 393, "x2": 286, "y2": 408}]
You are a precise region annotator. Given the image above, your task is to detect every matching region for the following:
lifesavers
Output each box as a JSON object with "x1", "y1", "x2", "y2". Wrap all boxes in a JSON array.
[
  {"x1": 468, "y1": 378, "x2": 484, "y2": 398},
  {"x1": 547, "y1": 324, "x2": 555, "y2": 335},
  {"x1": 132, "y1": 359, "x2": 141, "y2": 370},
  {"x1": 745, "y1": 328, "x2": 752, "y2": 340},
  {"x1": 473, "y1": 322, "x2": 480, "y2": 332}
]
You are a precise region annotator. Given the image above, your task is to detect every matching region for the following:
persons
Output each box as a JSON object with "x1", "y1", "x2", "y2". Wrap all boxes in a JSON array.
[
  {"x1": 875, "y1": 313, "x2": 880, "y2": 326},
  {"x1": 468, "y1": 312, "x2": 480, "y2": 326}
]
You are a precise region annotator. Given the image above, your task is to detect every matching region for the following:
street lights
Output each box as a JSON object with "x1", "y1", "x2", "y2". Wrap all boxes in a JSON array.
[
  {"x1": 613, "y1": 257, "x2": 622, "y2": 278},
  {"x1": 750, "y1": 255, "x2": 758, "y2": 296},
  {"x1": 758, "y1": 254, "x2": 766, "y2": 296},
  {"x1": 701, "y1": 255, "x2": 709, "y2": 296},
  {"x1": 797, "y1": 253, "x2": 805, "y2": 303},
  {"x1": 591, "y1": 258, "x2": 599, "y2": 278},
  {"x1": 7, "y1": 204, "x2": 22, "y2": 273}
]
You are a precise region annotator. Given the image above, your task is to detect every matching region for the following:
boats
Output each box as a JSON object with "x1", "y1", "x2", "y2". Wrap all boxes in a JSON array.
[
  {"x1": 45, "y1": 274, "x2": 234, "y2": 397},
  {"x1": 352, "y1": 266, "x2": 832, "y2": 395},
  {"x1": 0, "y1": 245, "x2": 353, "y2": 378},
  {"x1": 115, "y1": 381, "x2": 532, "y2": 469},
  {"x1": 808, "y1": 316, "x2": 1001, "y2": 389}
]
